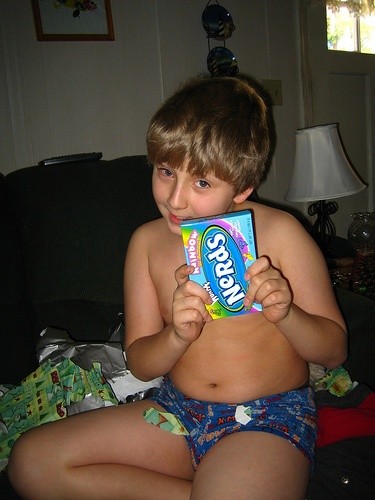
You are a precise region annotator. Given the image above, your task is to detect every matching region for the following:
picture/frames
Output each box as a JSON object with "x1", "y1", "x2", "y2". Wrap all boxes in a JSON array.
[{"x1": 33, "y1": 0, "x2": 114, "y2": 42}]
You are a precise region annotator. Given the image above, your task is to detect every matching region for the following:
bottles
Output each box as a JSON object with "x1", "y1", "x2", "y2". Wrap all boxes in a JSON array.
[{"x1": 347, "y1": 211, "x2": 375, "y2": 289}]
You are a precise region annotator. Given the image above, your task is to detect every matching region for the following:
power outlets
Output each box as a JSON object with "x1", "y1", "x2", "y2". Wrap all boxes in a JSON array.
[{"x1": 261, "y1": 80, "x2": 283, "y2": 107}]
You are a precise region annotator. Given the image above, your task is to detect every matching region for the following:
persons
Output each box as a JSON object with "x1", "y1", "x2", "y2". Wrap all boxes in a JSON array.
[{"x1": 8, "y1": 73, "x2": 349, "y2": 500}]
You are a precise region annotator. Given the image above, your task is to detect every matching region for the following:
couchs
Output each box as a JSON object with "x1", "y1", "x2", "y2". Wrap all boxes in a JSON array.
[{"x1": 0, "y1": 156, "x2": 375, "y2": 500}]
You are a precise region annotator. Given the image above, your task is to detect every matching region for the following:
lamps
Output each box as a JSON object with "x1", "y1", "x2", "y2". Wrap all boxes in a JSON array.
[{"x1": 284, "y1": 123, "x2": 367, "y2": 262}]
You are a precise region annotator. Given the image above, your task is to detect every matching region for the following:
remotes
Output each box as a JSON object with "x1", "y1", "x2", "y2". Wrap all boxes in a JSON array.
[{"x1": 38, "y1": 152, "x2": 102, "y2": 166}]
